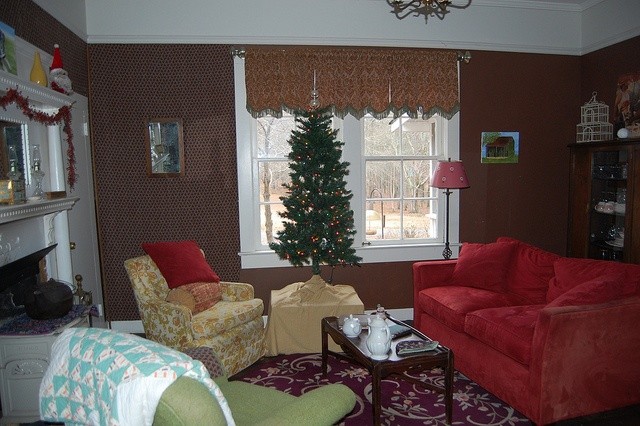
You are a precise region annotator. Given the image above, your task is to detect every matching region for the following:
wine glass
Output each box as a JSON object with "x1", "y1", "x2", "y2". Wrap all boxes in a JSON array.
[
  {"x1": 597, "y1": 163, "x2": 627, "y2": 177},
  {"x1": 609, "y1": 223, "x2": 624, "y2": 240}
]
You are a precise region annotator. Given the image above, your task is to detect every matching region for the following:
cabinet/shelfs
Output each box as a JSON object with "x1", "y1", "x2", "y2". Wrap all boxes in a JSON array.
[
  {"x1": 565, "y1": 138, "x2": 639, "y2": 265},
  {"x1": 1, "y1": 304, "x2": 92, "y2": 426}
]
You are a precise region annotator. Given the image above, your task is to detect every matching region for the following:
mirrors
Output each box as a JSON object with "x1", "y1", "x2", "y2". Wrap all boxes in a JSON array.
[
  {"x1": 0, "y1": 120, "x2": 24, "y2": 184},
  {"x1": 145, "y1": 117, "x2": 185, "y2": 177}
]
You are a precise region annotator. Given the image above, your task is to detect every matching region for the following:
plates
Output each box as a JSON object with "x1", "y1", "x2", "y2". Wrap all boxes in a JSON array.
[{"x1": 605, "y1": 237, "x2": 624, "y2": 248}]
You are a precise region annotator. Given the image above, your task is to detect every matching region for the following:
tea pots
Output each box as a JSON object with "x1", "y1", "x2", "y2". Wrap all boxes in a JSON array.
[
  {"x1": 366, "y1": 316, "x2": 392, "y2": 361},
  {"x1": 342, "y1": 314, "x2": 362, "y2": 338},
  {"x1": 22, "y1": 277, "x2": 73, "y2": 321}
]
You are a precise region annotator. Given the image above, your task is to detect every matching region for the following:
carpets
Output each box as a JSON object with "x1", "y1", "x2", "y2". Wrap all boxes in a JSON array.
[{"x1": 228, "y1": 320, "x2": 539, "y2": 426}]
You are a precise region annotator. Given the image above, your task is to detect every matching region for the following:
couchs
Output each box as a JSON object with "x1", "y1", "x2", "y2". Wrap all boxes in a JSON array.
[
  {"x1": 123, "y1": 248, "x2": 270, "y2": 381},
  {"x1": 48, "y1": 327, "x2": 357, "y2": 425},
  {"x1": 409, "y1": 235, "x2": 640, "y2": 425}
]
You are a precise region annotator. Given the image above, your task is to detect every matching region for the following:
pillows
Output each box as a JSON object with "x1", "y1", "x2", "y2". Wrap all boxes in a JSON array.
[
  {"x1": 142, "y1": 240, "x2": 221, "y2": 289},
  {"x1": 448, "y1": 241, "x2": 517, "y2": 292},
  {"x1": 528, "y1": 268, "x2": 627, "y2": 332}
]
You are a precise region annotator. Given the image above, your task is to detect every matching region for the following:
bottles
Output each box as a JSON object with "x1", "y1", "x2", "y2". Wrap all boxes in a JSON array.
[{"x1": 376, "y1": 307, "x2": 385, "y2": 320}]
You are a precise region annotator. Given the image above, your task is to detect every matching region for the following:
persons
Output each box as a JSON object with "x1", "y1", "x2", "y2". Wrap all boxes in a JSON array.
[{"x1": 49, "y1": 43, "x2": 73, "y2": 95}]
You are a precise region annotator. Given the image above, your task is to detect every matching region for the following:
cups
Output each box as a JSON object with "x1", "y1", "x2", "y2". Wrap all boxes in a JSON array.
[
  {"x1": 600, "y1": 203, "x2": 614, "y2": 213},
  {"x1": 594, "y1": 202, "x2": 605, "y2": 212}
]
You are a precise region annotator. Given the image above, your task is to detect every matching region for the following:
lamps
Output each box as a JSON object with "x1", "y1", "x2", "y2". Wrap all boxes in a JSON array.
[
  {"x1": 427, "y1": 157, "x2": 472, "y2": 261},
  {"x1": 385, "y1": 0, "x2": 473, "y2": 25}
]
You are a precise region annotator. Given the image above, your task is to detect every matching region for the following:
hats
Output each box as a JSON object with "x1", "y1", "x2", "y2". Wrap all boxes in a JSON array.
[{"x1": 48, "y1": 43, "x2": 68, "y2": 75}]
